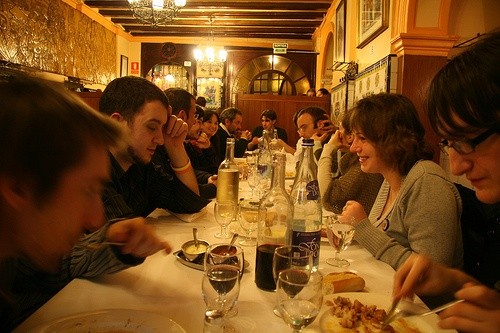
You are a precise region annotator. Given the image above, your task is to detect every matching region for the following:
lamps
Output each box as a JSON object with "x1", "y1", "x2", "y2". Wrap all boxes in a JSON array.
[
  {"x1": 193, "y1": 17, "x2": 229, "y2": 67},
  {"x1": 163, "y1": 62, "x2": 176, "y2": 84},
  {"x1": 128, "y1": 0, "x2": 187, "y2": 29}
]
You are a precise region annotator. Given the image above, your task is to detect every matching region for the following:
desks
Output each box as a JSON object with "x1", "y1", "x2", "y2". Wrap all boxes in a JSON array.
[{"x1": 11, "y1": 154, "x2": 458, "y2": 333}]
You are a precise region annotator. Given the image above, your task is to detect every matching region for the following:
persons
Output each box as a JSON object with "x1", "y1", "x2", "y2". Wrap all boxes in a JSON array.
[
  {"x1": 393, "y1": 31, "x2": 500, "y2": 333},
  {"x1": 0, "y1": 68, "x2": 385, "y2": 333},
  {"x1": 319, "y1": 93, "x2": 464, "y2": 311}
]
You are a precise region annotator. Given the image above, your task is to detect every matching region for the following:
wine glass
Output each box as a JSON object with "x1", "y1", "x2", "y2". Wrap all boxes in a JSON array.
[
  {"x1": 213, "y1": 199, "x2": 236, "y2": 239},
  {"x1": 272, "y1": 245, "x2": 314, "y2": 318},
  {"x1": 246, "y1": 173, "x2": 260, "y2": 200},
  {"x1": 201, "y1": 265, "x2": 241, "y2": 333},
  {"x1": 325, "y1": 214, "x2": 356, "y2": 269},
  {"x1": 247, "y1": 155, "x2": 257, "y2": 174},
  {"x1": 237, "y1": 201, "x2": 267, "y2": 247},
  {"x1": 204, "y1": 243, "x2": 244, "y2": 317},
  {"x1": 274, "y1": 268, "x2": 324, "y2": 333}
]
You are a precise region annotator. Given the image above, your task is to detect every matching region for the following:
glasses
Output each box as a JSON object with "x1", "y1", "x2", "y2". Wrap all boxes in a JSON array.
[
  {"x1": 296, "y1": 126, "x2": 307, "y2": 137},
  {"x1": 261, "y1": 120, "x2": 270, "y2": 123},
  {"x1": 193, "y1": 114, "x2": 199, "y2": 119},
  {"x1": 437, "y1": 117, "x2": 500, "y2": 156}
]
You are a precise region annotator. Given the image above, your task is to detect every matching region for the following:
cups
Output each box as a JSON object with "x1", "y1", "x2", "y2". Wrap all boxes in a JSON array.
[{"x1": 180, "y1": 239, "x2": 210, "y2": 262}]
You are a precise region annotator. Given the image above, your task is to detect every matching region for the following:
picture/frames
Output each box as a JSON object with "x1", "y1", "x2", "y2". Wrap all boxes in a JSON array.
[
  {"x1": 334, "y1": 0, "x2": 347, "y2": 66},
  {"x1": 121, "y1": 55, "x2": 129, "y2": 77},
  {"x1": 356, "y1": 0, "x2": 390, "y2": 48}
]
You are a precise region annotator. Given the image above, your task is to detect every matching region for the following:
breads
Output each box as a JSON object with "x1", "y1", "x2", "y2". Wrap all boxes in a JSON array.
[{"x1": 322, "y1": 271, "x2": 366, "y2": 296}]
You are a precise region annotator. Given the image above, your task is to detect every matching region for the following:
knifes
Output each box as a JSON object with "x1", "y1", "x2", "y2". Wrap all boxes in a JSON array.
[{"x1": 383, "y1": 296, "x2": 402, "y2": 327}]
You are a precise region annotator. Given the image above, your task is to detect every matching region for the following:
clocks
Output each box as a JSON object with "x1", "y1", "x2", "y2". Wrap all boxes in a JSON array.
[{"x1": 161, "y1": 42, "x2": 178, "y2": 58}]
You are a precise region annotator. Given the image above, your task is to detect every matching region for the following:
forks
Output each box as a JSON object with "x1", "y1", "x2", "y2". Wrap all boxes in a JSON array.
[{"x1": 381, "y1": 299, "x2": 465, "y2": 332}]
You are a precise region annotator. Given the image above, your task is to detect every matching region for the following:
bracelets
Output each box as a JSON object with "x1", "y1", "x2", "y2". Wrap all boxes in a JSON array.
[
  {"x1": 170, "y1": 156, "x2": 191, "y2": 171},
  {"x1": 174, "y1": 162, "x2": 192, "y2": 174}
]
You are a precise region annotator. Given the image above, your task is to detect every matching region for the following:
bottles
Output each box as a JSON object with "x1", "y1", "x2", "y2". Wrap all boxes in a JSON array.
[
  {"x1": 202, "y1": 309, "x2": 224, "y2": 333},
  {"x1": 255, "y1": 154, "x2": 294, "y2": 292},
  {"x1": 290, "y1": 139, "x2": 322, "y2": 273},
  {"x1": 215, "y1": 137, "x2": 240, "y2": 220},
  {"x1": 257, "y1": 130, "x2": 273, "y2": 188}
]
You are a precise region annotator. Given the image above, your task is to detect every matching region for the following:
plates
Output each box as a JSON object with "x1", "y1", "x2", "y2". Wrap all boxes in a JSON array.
[
  {"x1": 318, "y1": 307, "x2": 358, "y2": 333},
  {"x1": 39, "y1": 308, "x2": 187, "y2": 333},
  {"x1": 171, "y1": 250, "x2": 250, "y2": 271}
]
